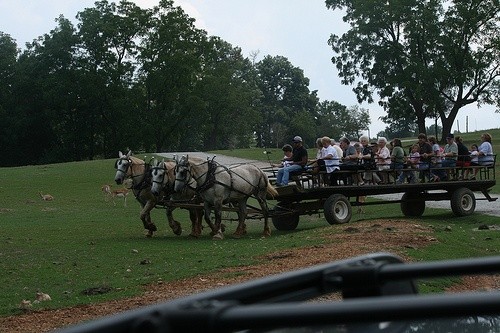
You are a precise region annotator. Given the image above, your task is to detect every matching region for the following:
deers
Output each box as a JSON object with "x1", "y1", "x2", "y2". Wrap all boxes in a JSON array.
[
  {"x1": 100, "y1": 183, "x2": 132, "y2": 207},
  {"x1": 37, "y1": 190, "x2": 55, "y2": 201}
]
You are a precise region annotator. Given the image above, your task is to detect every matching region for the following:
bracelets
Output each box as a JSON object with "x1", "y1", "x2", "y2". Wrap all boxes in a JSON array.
[
  {"x1": 446, "y1": 153, "x2": 447, "y2": 156},
  {"x1": 349, "y1": 155, "x2": 351, "y2": 160}
]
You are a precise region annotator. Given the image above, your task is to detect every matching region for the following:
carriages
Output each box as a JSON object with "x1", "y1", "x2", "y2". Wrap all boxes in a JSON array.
[{"x1": 113, "y1": 148, "x2": 498, "y2": 241}]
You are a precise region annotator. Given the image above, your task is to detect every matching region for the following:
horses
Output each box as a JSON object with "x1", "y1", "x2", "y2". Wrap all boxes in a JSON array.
[
  {"x1": 150, "y1": 158, "x2": 228, "y2": 240},
  {"x1": 114, "y1": 148, "x2": 205, "y2": 237},
  {"x1": 172, "y1": 153, "x2": 280, "y2": 242}
]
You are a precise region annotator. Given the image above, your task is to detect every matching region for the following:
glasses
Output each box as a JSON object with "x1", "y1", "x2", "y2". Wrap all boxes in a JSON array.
[{"x1": 293, "y1": 141, "x2": 299, "y2": 144}]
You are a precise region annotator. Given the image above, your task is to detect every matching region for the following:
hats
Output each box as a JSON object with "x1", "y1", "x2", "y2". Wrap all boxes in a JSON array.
[
  {"x1": 427, "y1": 136, "x2": 435, "y2": 141},
  {"x1": 446, "y1": 134, "x2": 454, "y2": 139}
]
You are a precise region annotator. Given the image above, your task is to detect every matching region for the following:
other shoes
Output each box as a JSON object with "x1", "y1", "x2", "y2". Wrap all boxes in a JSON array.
[
  {"x1": 275, "y1": 182, "x2": 288, "y2": 186},
  {"x1": 433, "y1": 176, "x2": 438, "y2": 182},
  {"x1": 470, "y1": 174, "x2": 475, "y2": 180}
]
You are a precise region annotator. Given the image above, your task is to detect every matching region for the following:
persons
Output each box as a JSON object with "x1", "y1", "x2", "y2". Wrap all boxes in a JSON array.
[
  {"x1": 272, "y1": 136, "x2": 308, "y2": 186},
  {"x1": 463, "y1": 132, "x2": 493, "y2": 181},
  {"x1": 312, "y1": 136, "x2": 358, "y2": 186},
  {"x1": 272, "y1": 144, "x2": 302, "y2": 187},
  {"x1": 437, "y1": 134, "x2": 471, "y2": 181},
  {"x1": 354, "y1": 135, "x2": 407, "y2": 186},
  {"x1": 396, "y1": 132, "x2": 441, "y2": 184}
]
